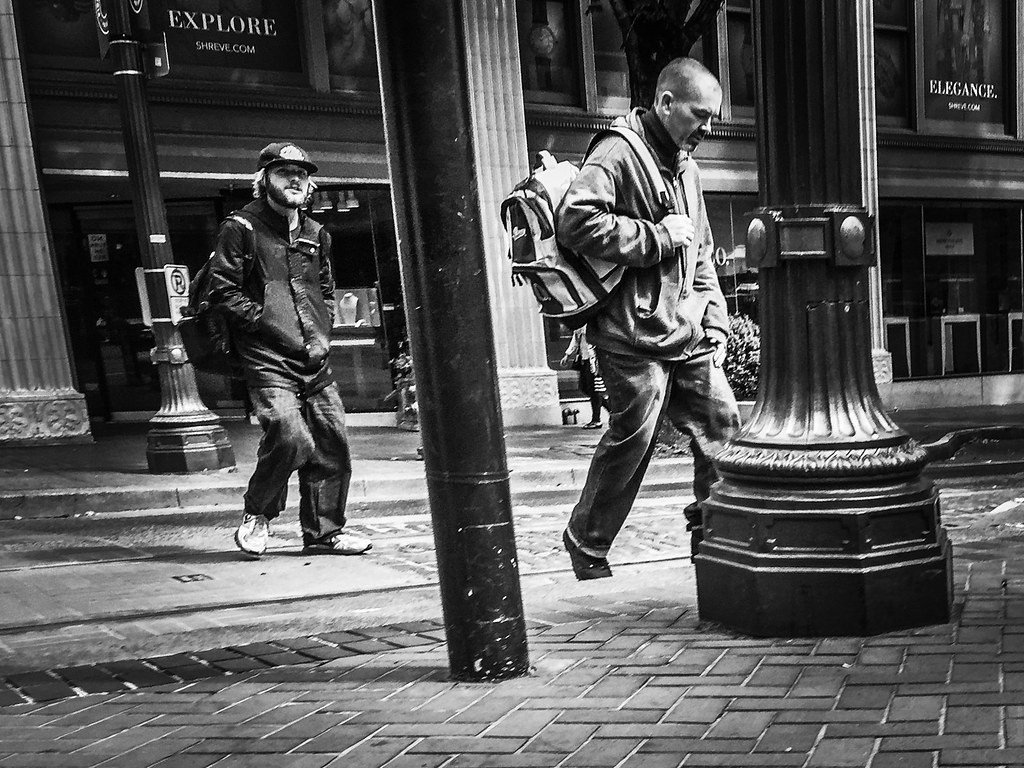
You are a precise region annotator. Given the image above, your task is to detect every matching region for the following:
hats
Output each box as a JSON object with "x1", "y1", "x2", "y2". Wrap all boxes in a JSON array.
[{"x1": 258, "y1": 139, "x2": 319, "y2": 176}]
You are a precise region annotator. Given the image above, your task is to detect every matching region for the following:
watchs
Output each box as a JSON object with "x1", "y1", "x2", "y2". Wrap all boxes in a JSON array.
[{"x1": 528, "y1": 0, "x2": 555, "y2": 92}]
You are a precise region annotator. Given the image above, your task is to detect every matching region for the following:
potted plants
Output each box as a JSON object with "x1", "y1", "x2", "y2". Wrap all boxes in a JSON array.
[{"x1": 721, "y1": 311, "x2": 762, "y2": 426}]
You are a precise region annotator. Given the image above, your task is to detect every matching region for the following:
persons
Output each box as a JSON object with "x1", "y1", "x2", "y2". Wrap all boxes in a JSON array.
[
  {"x1": 205, "y1": 142, "x2": 373, "y2": 556},
  {"x1": 559, "y1": 57, "x2": 742, "y2": 581}
]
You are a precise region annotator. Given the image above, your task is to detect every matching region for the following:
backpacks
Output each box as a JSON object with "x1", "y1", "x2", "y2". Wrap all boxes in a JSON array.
[
  {"x1": 500, "y1": 127, "x2": 677, "y2": 331},
  {"x1": 179, "y1": 216, "x2": 257, "y2": 376}
]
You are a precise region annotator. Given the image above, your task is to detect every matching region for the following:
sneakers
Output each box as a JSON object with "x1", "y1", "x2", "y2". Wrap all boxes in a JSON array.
[
  {"x1": 234, "y1": 512, "x2": 274, "y2": 559},
  {"x1": 301, "y1": 533, "x2": 373, "y2": 554},
  {"x1": 562, "y1": 527, "x2": 613, "y2": 580}
]
